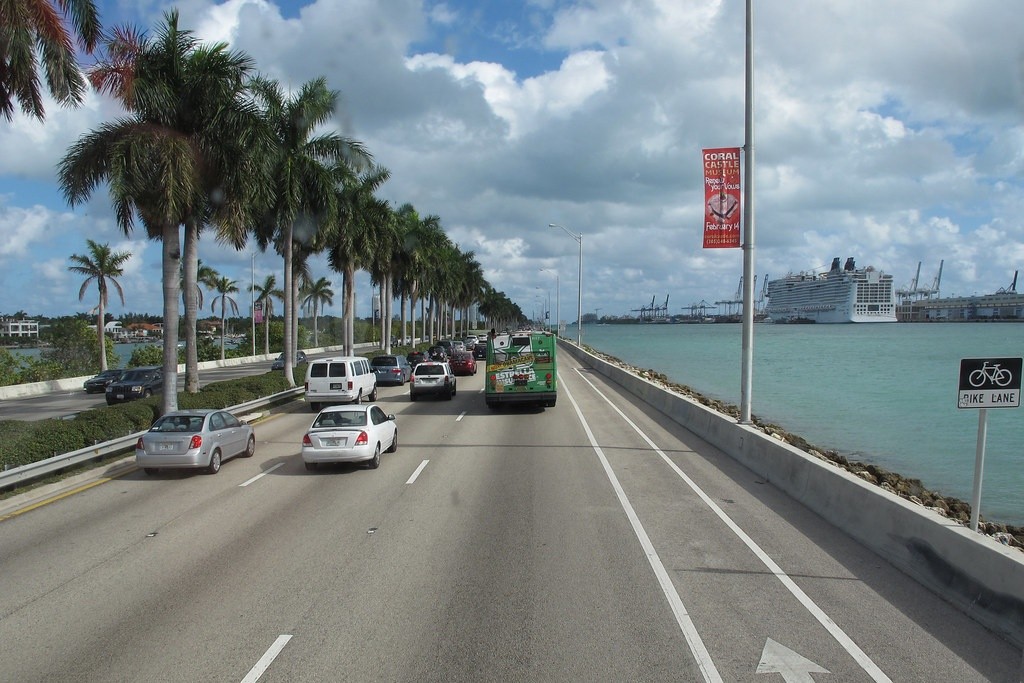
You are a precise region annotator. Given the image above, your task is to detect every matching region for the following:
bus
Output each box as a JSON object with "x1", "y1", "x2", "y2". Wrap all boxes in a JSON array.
[{"x1": 485, "y1": 329, "x2": 558, "y2": 407}]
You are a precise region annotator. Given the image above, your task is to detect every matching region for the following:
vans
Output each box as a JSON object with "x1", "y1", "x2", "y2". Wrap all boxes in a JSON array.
[{"x1": 302, "y1": 355, "x2": 377, "y2": 408}]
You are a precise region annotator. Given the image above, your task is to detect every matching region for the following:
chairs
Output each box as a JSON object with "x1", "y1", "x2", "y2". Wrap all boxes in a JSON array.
[
  {"x1": 163, "y1": 422, "x2": 188, "y2": 430},
  {"x1": 323, "y1": 420, "x2": 334, "y2": 426}
]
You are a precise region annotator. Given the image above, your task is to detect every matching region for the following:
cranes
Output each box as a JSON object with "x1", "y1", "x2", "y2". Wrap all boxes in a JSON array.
[
  {"x1": 930, "y1": 259, "x2": 944, "y2": 290},
  {"x1": 759, "y1": 272, "x2": 768, "y2": 301},
  {"x1": 733, "y1": 276, "x2": 743, "y2": 301},
  {"x1": 753, "y1": 275, "x2": 759, "y2": 290},
  {"x1": 909, "y1": 261, "x2": 922, "y2": 292},
  {"x1": 660, "y1": 294, "x2": 670, "y2": 309},
  {"x1": 645, "y1": 295, "x2": 655, "y2": 310}
]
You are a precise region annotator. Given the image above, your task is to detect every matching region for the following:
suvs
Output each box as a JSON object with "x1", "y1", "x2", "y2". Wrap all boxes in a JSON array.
[
  {"x1": 104, "y1": 365, "x2": 163, "y2": 407},
  {"x1": 409, "y1": 361, "x2": 456, "y2": 401}
]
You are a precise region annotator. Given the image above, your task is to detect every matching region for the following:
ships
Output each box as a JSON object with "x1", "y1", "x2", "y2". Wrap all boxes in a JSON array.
[{"x1": 763, "y1": 256, "x2": 899, "y2": 324}]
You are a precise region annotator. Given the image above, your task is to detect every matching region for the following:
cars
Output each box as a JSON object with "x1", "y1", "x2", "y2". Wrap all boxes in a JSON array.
[
  {"x1": 472, "y1": 343, "x2": 486, "y2": 360},
  {"x1": 407, "y1": 350, "x2": 433, "y2": 369},
  {"x1": 433, "y1": 330, "x2": 507, "y2": 355},
  {"x1": 398, "y1": 336, "x2": 412, "y2": 346},
  {"x1": 427, "y1": 346, "x2": 448, "y2": 363},
  {"x1": 271, "y1": 350, "x2": 308, "y2": 371},
  {"x1": 370, "y1": 355, "x2": 413, "y2": 386},
  {"x1": 134, "y1": 408, "x2": 257, "y2": 474},
  {"x1": 83, "y1": 367, "x2": 127, "y2": 393},
  {"x1": 302, "y1": 404, "x2": 398, "y2": 471},
  {"x1": 390, "y1": 335, "x2": 398, "y2": 348},
  {"x1": 449, "y1": 351, "x2": 477, "y2": 376}
]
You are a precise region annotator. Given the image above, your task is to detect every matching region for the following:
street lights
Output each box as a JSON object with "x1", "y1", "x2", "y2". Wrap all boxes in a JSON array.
[
  {"x1": 539, "y1": 267, "x2": 560, "y2": 339},
  {"x1": 548, "y1": 223, "x2": 582, "y2": 347},
  {"x1": 535, "y1": 286, "x2": 552, "y2": 333}
]
